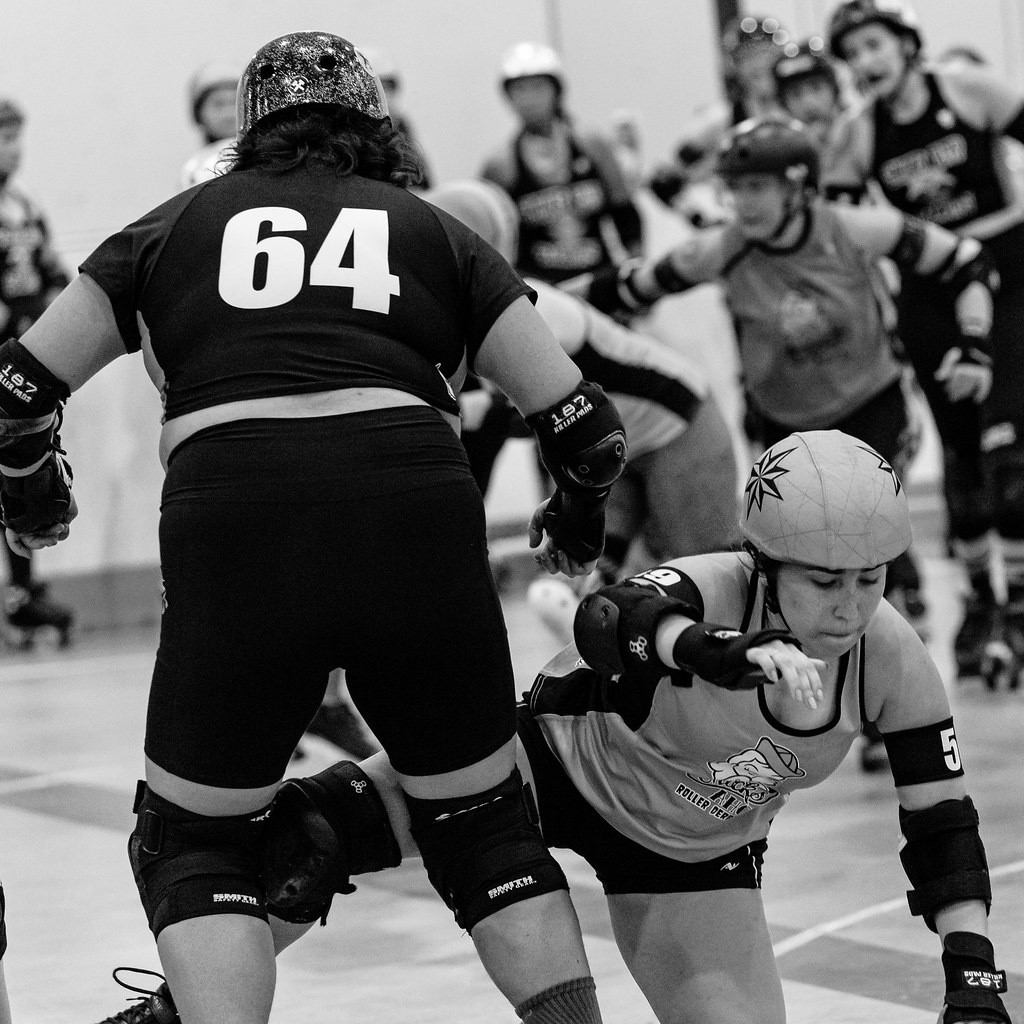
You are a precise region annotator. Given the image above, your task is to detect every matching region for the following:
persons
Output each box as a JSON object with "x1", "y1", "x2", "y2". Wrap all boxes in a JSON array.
[{"x1": 0, "y1": 2, "x2": 1024, "y2": 1023}]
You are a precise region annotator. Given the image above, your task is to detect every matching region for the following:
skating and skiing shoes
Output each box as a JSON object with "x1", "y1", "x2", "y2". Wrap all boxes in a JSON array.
[{"x1": 2, "y1": 582, "x2": 76, "y2": 646}]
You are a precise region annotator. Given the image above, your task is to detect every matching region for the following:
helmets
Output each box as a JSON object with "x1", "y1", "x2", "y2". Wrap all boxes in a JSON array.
[
  {"x1": 710, "y1": 113, "x2": 823, "y2": 191},
  {"x1": 739, "y1": 429, "x2": 913, "y2": 571},
  {"x1": 191, "y1": 61, "x2": 242, "y2": 121},
  {"x1": 499, "y1": 42, "x2": 570, "y2": 100},
  {"x1": 731, "y1": 20, "x2": 790, "y2": 65},
  {"x1": 770, "y1": 44, "x2": 841, "y2": 112},
  {"x1": 236, "y1": 33, "x2": 392, "y2": 146},
  {"x1": 825, "y1": 0, "x2": 923, "y2": 62}
]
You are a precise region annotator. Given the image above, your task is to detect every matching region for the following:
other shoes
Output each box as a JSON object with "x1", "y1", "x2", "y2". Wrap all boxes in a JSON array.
[{"x1": 953, "y1": 589, "x2": 1024, "y2": 692}]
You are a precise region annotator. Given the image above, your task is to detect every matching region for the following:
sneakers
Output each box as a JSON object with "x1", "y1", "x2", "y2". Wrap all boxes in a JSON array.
[{"x1": 96, "y1": 982, "x2": 182, "y2": 1024}]
